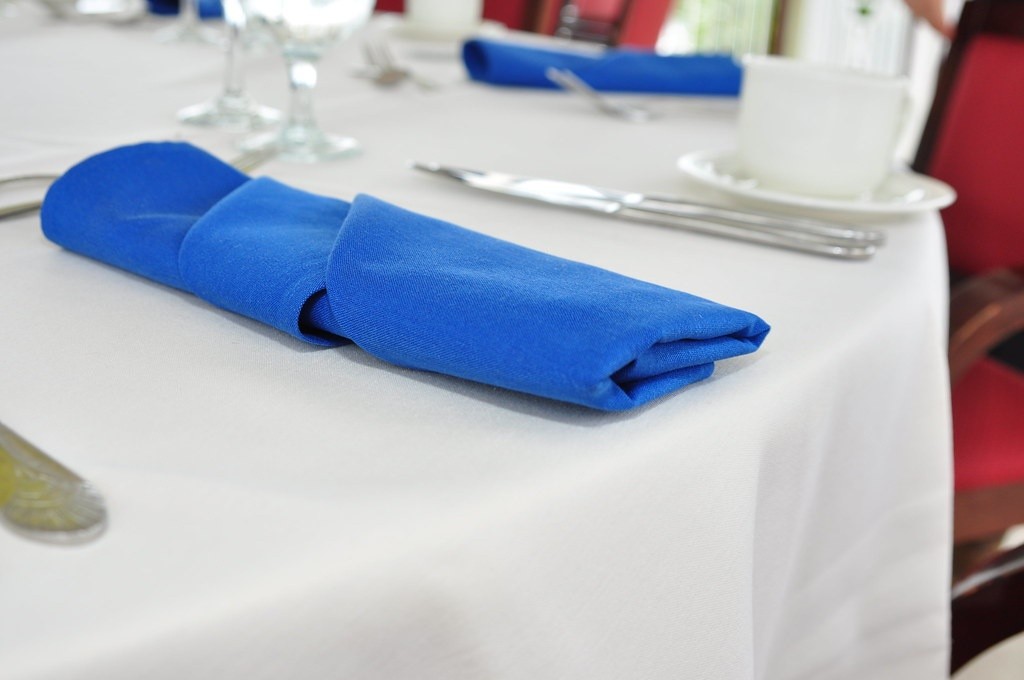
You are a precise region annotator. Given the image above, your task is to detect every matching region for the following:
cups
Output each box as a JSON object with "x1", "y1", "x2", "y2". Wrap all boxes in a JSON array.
[{"x1": 737, "y1": 54, "x2": 903, "y2": 199}]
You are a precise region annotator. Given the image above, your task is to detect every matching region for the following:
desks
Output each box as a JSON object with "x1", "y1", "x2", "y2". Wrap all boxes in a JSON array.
[{"x1": 0, "y1": 0, "x2": 947, "y2": 680}]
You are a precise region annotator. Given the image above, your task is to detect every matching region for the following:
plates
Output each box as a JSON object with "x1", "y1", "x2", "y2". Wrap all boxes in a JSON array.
[{"x1": 681, "y1": 146, "x2": 958, "y2": 214}]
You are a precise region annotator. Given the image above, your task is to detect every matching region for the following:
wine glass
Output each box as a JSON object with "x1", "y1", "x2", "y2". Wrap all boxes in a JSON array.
[
  {"x1": 239, "y1": 0, "x2": 375, "y2": 160},
  {"x1": 177, "y1": 0, "x2": 254, "y2": 127}
]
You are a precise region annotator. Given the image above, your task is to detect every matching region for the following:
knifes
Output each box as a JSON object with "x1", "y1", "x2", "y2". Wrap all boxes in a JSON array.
[{"x1": 412, "y1": 162, "x2": 883, "y2": 260}]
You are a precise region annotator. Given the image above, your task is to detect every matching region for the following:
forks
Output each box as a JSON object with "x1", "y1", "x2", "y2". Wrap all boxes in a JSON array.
[{"x1": 547, "y1": 67, "x2": 737, "y2": 123}]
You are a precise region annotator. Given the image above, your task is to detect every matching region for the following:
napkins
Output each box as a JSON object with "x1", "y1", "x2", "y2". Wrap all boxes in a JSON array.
[
  {"x1": 40, "y1": 140, "x2": 769, "y2": 418},
  {"x1": 460, "y1": 37, "x2": 745, "y2": 100}
]
